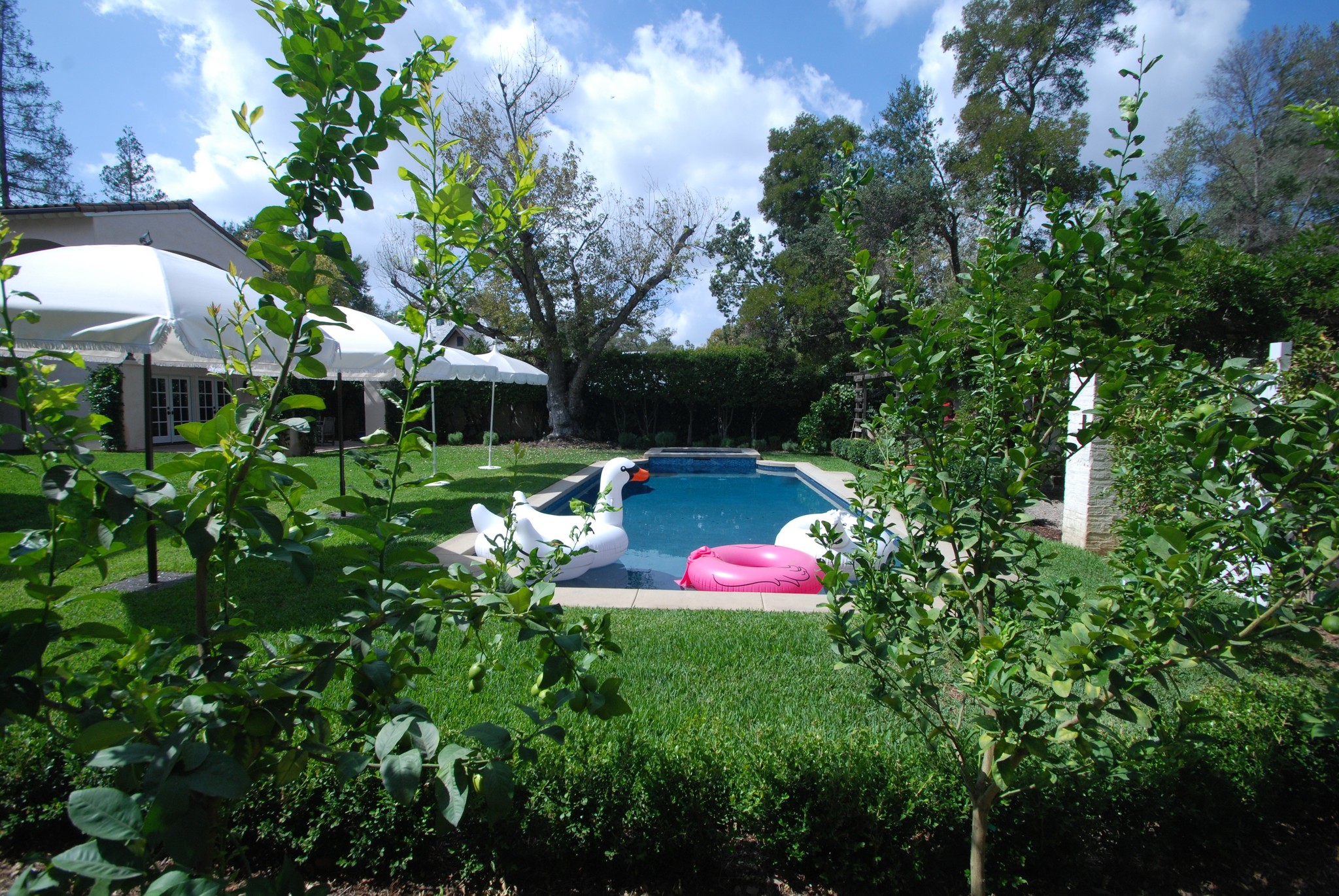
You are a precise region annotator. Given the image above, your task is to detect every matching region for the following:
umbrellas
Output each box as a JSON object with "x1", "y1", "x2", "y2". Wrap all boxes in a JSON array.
[
  {"x1": 421, "y1": 332, "x2": 499, "y2": 469},
  {"x1": 0, "y1": 231, "x2": 400, "y2": 582},
  {"x1": 207, "y1": 306, "x2": 450, "y2": 514},
  {"x1": 473, "y1": 343, "x2": 549, "y2": 466}
]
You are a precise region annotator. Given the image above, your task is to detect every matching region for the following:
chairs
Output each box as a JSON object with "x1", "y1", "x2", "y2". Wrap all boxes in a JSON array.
[{"x1": 317, "y1": 417, "x2": 336, "y2": 446}]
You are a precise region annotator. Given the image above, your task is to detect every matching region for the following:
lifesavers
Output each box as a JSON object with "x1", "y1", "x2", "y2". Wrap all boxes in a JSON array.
[
  {"x1": 688, "y1": 544, "x2": 825, "y2": 594},
  {"x1": 774, "y1": 509, "x2": 894, "y2": 581},
  {"x1": 471, "y1": 457, "x2": 650, "y2": 586}
]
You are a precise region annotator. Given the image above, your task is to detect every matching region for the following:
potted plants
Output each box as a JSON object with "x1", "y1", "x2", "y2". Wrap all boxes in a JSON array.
[{"x1": 883, "y1": 454, "x2": 921, "y2": 486}]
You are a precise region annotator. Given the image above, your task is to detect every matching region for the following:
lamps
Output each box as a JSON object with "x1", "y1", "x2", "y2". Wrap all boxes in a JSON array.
[{"x1": 140, "y1": 230, "x2": 154, "y2": 246}]
